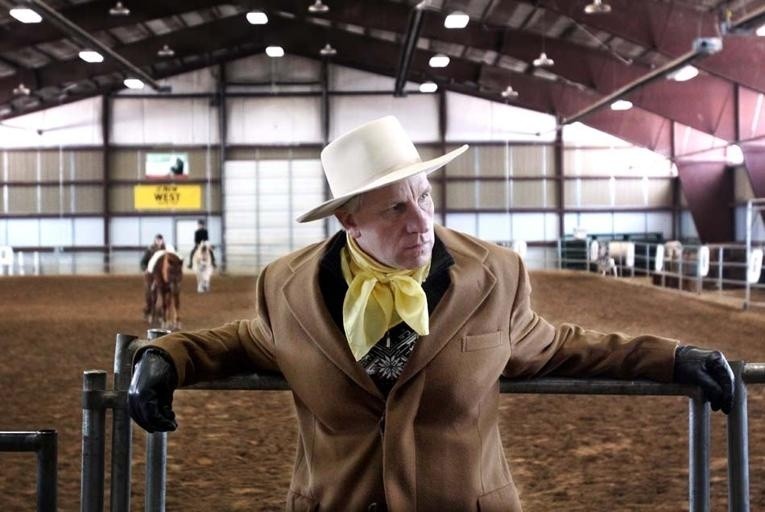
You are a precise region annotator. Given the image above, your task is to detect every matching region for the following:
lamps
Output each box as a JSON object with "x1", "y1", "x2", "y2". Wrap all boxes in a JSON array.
[
  {"x1": 119, "y1": 76, "x2": 148, "y2": 94},
  {"x1": 501, "y1": 85, "x2": 519, "y2": 101},
  {"x1": 418, "y1": 79, "x2": 439, "y2": 97},
  {"x1": 755, "y1": 24, "x2": 764, "y2": 39},
  {"x1": 416, "y1": 2, "x2": 444, "y2": 15},
  {"x1": 265, "y1": 44, "x2": 287, "y2": 60},
  {"x1": 609, "y1": 97, "x2": 635, "y2": 112},
  {"x1": 534, "y1": 51, "x2": 557, "y2": 71},
  {"x1": 584, "y1": 3, "x2": 614, "y2": 18},
  {"x1": 75, "y1": 48, "x2": 105, "y2": 67},
  {"x1": 10, "y1": 4, "x2": 43, "y2": 26},
  {"x1": 243, "y1": 11, "x2": 271, "y2": 28},
  {"x1": 441, "y1": 9, "x2": 471, "y2": 32},
  {"x1": 111, "y1": 4, "x2": 131, "y2": 19},
  {"x1": 157, "y1": 44, "x2": 175, "y2": 58},
  {"x1": 310, "y1": 1, "x2": 329, "y2": 14},
  {"x1": 425, "y1": 52, "x2": 451, "y2": 70},
  {"x1": 666, "y1": 59, "x2": 701, "y2": 86},
  {"x1": 317, "y1": 43, "x2": 338, "y2": 60}
]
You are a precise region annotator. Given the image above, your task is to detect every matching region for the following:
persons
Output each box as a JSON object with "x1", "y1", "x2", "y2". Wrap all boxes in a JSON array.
[
  {"x1": 185, "y1": 218, "x2": 219, "y2": 271},
  {"x1": 138, "y1": 234, "x2": 167, "y2": 275},
  {"x1": 171, "y1": 155, "x2": 184, "y2": 176},
  {"x1": 123, "y1": 114, "x2": 738, "y2": 512}
]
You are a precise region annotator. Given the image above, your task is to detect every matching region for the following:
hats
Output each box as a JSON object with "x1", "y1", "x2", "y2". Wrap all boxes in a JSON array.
[{"x1": 298, "y1": 116, "x2": 469, "y2": 223}]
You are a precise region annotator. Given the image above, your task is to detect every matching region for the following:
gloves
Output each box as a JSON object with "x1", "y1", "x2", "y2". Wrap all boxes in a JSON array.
[
  {"x1": 128, "y1": 351, "x2": 177, "y2": 432},
  {"x1": 676, "y1": 345, "x2": 735, "y2": 413}
]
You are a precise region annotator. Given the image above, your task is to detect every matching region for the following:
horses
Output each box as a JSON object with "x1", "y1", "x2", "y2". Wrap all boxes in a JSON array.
[
  {"x1": 146, "y1": 249, "x2": 185, "y2": 331},
  {"x1": 192, "y1": 241, "x2": 212, "y2": 295}
]
